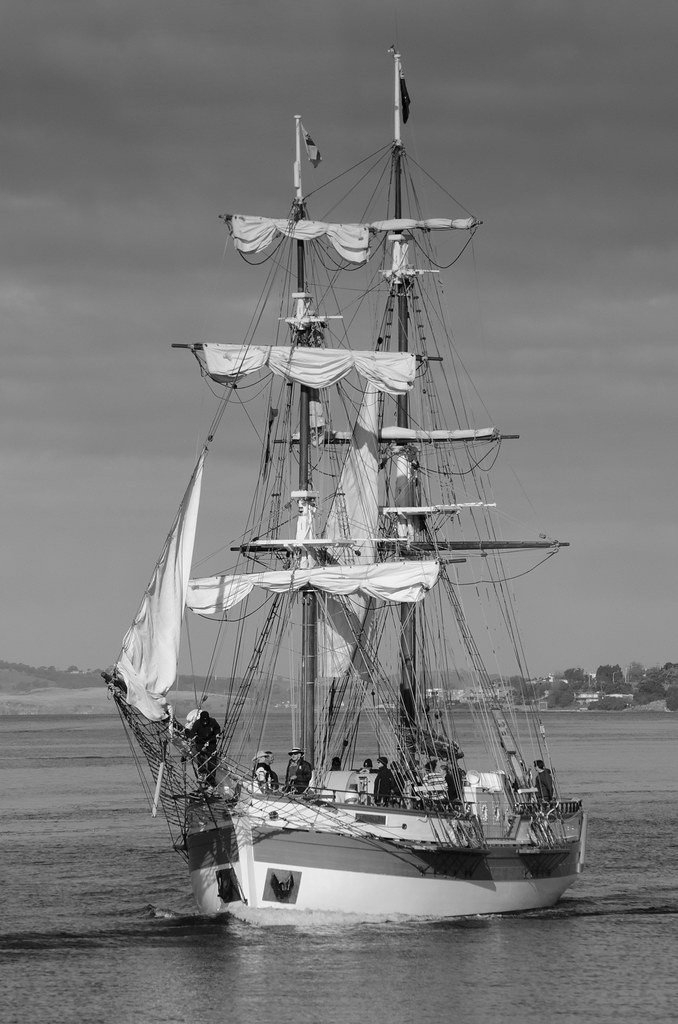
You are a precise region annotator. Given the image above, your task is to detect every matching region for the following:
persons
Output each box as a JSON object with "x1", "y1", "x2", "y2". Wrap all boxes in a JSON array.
[
  {"x1": 390, "y1": 760, "x2": 405, "y2": 805},
  {"x1": 185, "y1": 712, "x2": 222, "y2": 789},
  {"x1": 420, "y1": 760, "x2": 448, "y2": 807},
  {"x1": 403, "y1": 760, "x2": 423, "y2": 809},
  {"x1": 262, "y1": 750, "x2": 277, "y2": 790},
  {"x1": 285, "y1": 746, "x2": 313, "y2": 794},
  {"x1": 358, "y1": 759, "x2": 377, "y2": 772},
  {"x1": 253, "y1": 750, "x2": 273, "y2": 789},
  {"x1": 439, "y1": 764, "x2": 458, "y2": 807},
  {"x1": 328, "y1": 757, "x2": 342, "y2": 770},
  {"x1": 534, "y1": 760, "x2": 553, "y2": 811},
  {"x1": 374, "y1": 757, "x2": 393, "y2": 804}
]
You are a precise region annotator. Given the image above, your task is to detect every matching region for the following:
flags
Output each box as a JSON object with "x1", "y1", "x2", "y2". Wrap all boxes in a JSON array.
[
  {"x1": 301, "y1": 122, "x2": 323, "y2": 168},
  {"x1": 400, "y1": 58, "x2": 411, "y2": 123}
]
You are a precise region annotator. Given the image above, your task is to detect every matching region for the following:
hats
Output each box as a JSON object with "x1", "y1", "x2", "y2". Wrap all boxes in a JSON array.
[
  {"x1": 378, "y1": 757, "x2": 388, "y2": 766},
  {"x1": 363, "y1": 759, "x2": 372, "y2": 767},
  {"x1": 287, "y1": 748, "x2": 304, "y2": 757},
  {"x1": 252, "y1": 751, "x2": 270, "y2": 761}
]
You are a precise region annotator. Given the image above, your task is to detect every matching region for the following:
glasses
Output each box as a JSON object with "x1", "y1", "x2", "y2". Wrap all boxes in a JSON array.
[{"x1": 291, "y1": 753, "x2": 298, "y2": 755}]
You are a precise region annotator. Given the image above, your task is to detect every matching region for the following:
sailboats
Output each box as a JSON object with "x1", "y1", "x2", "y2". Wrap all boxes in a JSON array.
[{"x1": 101, "y1": 47, "x2": 587, "y2": 923}]
